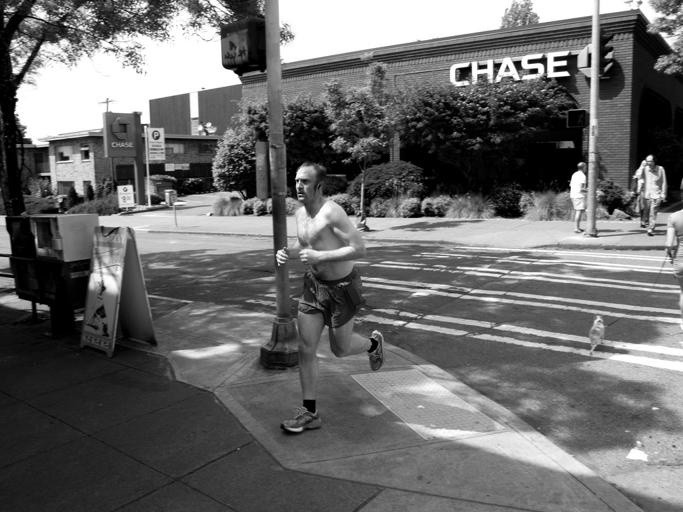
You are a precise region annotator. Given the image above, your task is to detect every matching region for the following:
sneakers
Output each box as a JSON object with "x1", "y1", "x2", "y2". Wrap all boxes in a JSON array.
[
  {"x1": 281, "y1": 410, "x2": 321, "y2": 432},
  {"x1": 368, "y1": 330, "x2": 384, "y2": 370}
]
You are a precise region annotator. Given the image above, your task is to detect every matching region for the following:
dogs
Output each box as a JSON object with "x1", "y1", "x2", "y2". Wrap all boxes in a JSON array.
[{"x1": 588, "y1": 315, "x2": 605, "y2": 355}]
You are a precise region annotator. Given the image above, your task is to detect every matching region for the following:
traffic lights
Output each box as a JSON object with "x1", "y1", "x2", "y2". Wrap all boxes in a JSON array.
[{"x1": 598, "y1": 33, "x2": 613, "y2": 75}]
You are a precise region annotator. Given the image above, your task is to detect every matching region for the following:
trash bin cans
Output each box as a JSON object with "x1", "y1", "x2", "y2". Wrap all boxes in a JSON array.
[{"x1": 144, "y1": 174, "x2": 177, "y2": 201}]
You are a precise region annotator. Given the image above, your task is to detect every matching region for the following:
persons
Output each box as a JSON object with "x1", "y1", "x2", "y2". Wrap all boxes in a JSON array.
[
  {"x1": 569, "y1": 162, "x2": 588, "y2": 232},
  {"x1": 664, "y1": 209, "x2": 682, "y2": 332},
  {"x1": 276, "y1": 161, "x2": 385, "y2": 432},
  {"x1": 633, "y1": 156, "x2": 667, "y2": 235}
]
